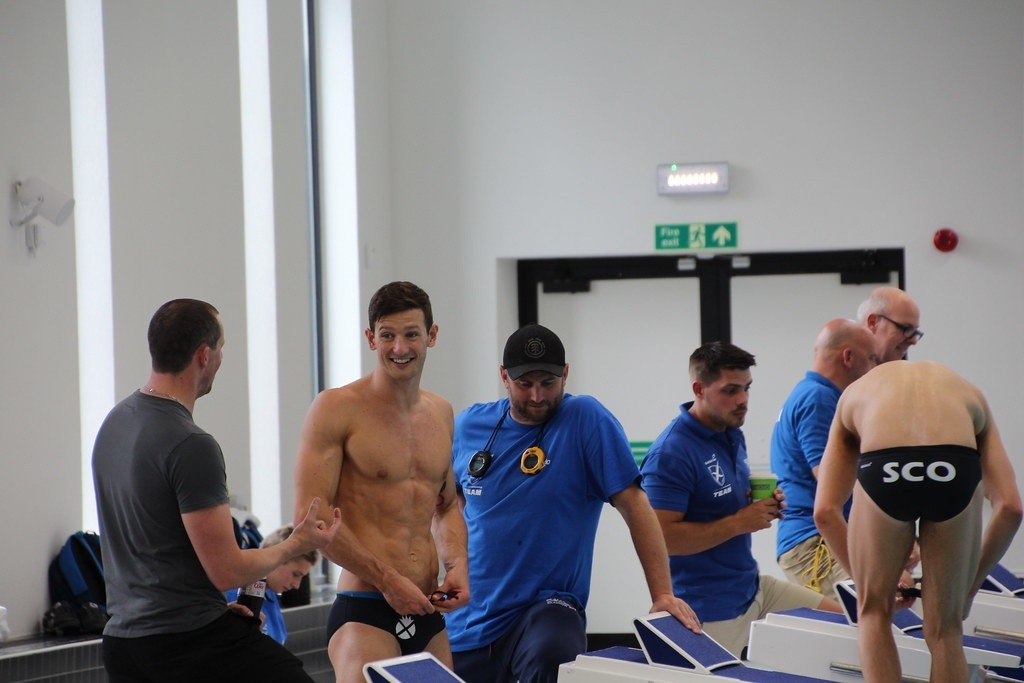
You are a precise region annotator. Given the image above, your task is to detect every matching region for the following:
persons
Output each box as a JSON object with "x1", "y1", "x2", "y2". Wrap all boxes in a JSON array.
[
  {"x1": 811, "y1": 361, "x2": 1023, "y2": 683},
  {"x1": 639, "y1": 340, "x2": 843, "y2": 661},
  {"x1": 769, "y1": 318, "x2": 918, "y2": 607},
  {"x1": 90, "y1": 297, "x2": 342, "y2": 683},
  {"x1": 856, "y1": 285, "x2": 920, "y2": 364},
  {"x1": 292, "y1": 282, "x2": 472, "y2": 683},
  {"x1": 442, "y1": 324, "x2": 703, "y2": 683},
  {"x1": 221, "y1": 523, "x2": 319, "y2": 646}
]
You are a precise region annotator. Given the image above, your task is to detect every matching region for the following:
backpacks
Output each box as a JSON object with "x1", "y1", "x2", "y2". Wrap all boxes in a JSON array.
[
  {"x1": 42, "y1": 530, "x2": 112, "y2": 639},
  {"x1": 240, "y1": 520, "x2": 311, "y2": 609}
]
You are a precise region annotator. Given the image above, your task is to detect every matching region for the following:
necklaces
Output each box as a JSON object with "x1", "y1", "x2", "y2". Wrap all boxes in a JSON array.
[{"x1": 141, "y1": 384, "x2": 186, "y2": 407}]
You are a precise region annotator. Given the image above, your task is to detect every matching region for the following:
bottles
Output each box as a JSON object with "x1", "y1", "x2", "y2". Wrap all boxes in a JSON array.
[{"x1": 237, "y1": 575, "x2": 267, "y2": 619}]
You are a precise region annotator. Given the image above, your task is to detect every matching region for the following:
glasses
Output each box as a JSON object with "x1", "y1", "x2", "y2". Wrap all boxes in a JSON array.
[{"x1": 878, "y1": 314, "x2": 924, "y2": 341}]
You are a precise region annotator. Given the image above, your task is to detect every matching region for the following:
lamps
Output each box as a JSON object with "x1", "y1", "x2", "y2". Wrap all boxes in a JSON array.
[{"x1": 9, "y1": 180, "x2": 75, "y2": 249}]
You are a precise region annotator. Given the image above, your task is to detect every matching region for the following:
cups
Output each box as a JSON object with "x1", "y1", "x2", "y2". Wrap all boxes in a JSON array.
[{"x1": 749, "y1": 475, "x2": 778, "y2": 503}]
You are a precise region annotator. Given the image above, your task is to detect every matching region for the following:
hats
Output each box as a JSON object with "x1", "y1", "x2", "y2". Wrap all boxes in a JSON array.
[{"x1": 502, "y1": 323, "x2": 566, "y2": 382}]
